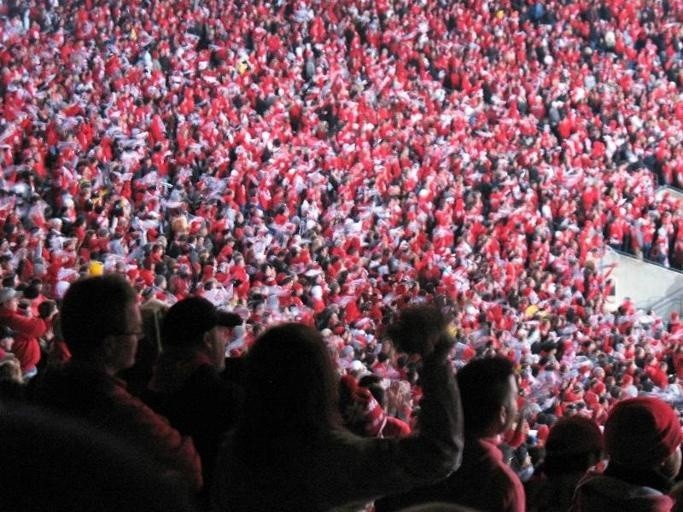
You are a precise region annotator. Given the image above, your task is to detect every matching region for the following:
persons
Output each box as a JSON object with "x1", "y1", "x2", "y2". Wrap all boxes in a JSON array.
[{"x1": 0, "y1": 1, "x2": 683, "y2": 512}]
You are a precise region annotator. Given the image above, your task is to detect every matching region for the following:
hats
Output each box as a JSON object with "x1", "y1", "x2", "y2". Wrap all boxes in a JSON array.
[
  {"x1": 604, "y1": 396, "x2": 683, "y2": 461},
  {"x1": 159, "y1": 295, "x2": 243, "y2": 347}
]
